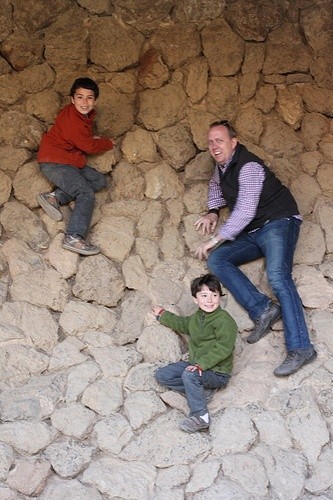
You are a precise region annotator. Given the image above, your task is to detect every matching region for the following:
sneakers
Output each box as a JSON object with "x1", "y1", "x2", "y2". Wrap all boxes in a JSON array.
[
  {"x1": 247, "y1": 300, "x2": 282, "y2": 344},
  {"x1": 37, "y1": 192, "x2": 63, "y2": 222},
  {"x1": 274, "y1": 347, "x2": 317, "y2": 377},
  {"x1": 179, "y1": 414, "x2": 210, "y2": 434},
  {"x1": 203, "y1": 387, "x2": 218, "y2": 403},
  {"x1": 62, "y1": 233, "x2": 100, "y2": 255}
]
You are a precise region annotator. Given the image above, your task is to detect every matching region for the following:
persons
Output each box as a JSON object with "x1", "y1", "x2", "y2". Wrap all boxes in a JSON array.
[
  {"x1": 194, "y1": 119, "x2": 317, "y2": 377},
  {"x1": 36, "y1": 76, "x2": 117, "y2": 256},
  {"x1": 153, "y1": 273, "x2": 238, "y2": 433}
]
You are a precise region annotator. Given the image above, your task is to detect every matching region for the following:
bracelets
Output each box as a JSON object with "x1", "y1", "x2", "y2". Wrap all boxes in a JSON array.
[{"x1": 210, "y1": 235, "x2": 219, "y2": 246}]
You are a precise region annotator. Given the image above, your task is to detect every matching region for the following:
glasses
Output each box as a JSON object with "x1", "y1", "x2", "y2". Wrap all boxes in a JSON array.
[{"x1": 210, "y1": 119, "x2": 232, "y2": 126}]
